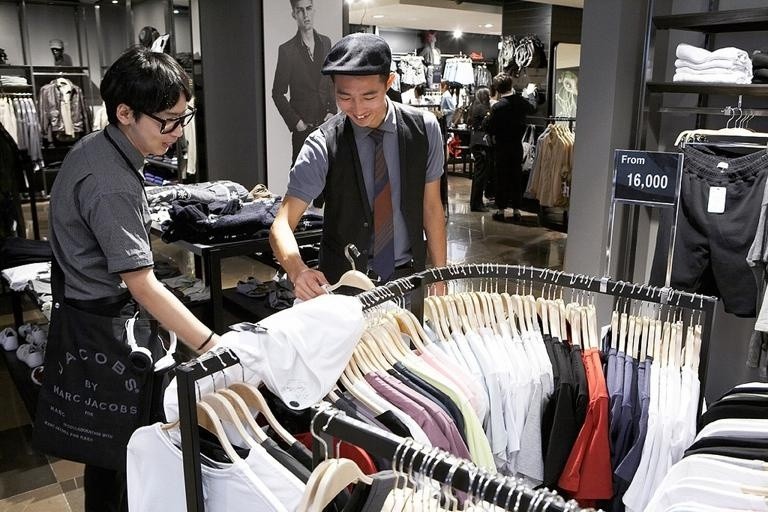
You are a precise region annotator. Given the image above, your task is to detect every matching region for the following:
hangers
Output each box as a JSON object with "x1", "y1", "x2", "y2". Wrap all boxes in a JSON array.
[
  {"x1": 140, "y1": 271, "x2": 424, "y2": 512},
  {"x1": 539, "y1": 116, "x2": 574, "y2": 150},
  {"x1": 294, "y1": 404, "x2": 600, "y2": 512},
  {"x1": 0, "y1": 92, "x2": 36, "y2": 115},
  {"x1": 674, "y1": 104, "x2": 768, "y2": 152},
  {"x1": 425, "y1": 262, "x2": 717, "y2": 417},
  {"x1": 41, "y1": 72, "x2": 80, "y2": 91}
]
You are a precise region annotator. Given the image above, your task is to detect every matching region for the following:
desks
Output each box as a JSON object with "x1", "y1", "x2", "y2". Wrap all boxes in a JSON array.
[{"x1": 143, "y1": 209, "x2": 324, "y2": 338}]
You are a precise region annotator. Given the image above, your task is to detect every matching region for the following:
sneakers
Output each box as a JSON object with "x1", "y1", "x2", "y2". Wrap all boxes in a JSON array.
[
  {"x1": 479, "y1": 206, "x2": 489, "y2": 212},
  {"x1": 492, "y1": 211, "x2": 504, "y2": 221}
]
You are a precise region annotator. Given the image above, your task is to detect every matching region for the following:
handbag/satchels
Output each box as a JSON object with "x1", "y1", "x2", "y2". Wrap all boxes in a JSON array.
[{"x1": 31, "y1": 260, "x2": 160, "y2": 470}]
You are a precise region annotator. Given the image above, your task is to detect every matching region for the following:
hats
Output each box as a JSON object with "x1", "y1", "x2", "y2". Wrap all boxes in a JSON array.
[
  {"x1": 49, "y1": 39, "x2": 64, "y2": 49},
  {"x1": 321, "y1": 32, "x2": 392, "y2": 76}
]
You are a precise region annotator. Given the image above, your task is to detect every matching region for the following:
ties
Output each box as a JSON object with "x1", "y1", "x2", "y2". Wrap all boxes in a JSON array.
[{"x1": 368, "y1": 129, "x2": 395, "y2": 282}]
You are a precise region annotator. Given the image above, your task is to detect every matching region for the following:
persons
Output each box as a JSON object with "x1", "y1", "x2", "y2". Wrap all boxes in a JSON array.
[
  {"x1": 483, "y1": 72, "x2": 538, "y2": 224},
  {"x1": 269, "y1": 32, "x2": 448, "y2": 313},
  {"x1": 470, "y1": 88, "x2": 490, "y2": 212},
  {"x1": 440, "y1": 81, "x2": 455, "y2": 110},
  {"x1": 420, "y1": 30, "x2": 442, "y2": 64},
  {"x1": 272, "y1": 0, "x2": 338, "y2": 215},
  {"x1": 489, "y1": 86, "x2": 498, "y2": 107},
  {"x1": 46, "y1": 46, "x2": 222, "y2": 512},
  {"x1": 410, "y1": 86, "x2": 427, "y2": 109}
]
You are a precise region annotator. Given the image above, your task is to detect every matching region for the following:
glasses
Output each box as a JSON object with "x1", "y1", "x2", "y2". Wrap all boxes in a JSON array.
[{"x1": 137, "y1": 104, "x2": 197, "y2": 134}]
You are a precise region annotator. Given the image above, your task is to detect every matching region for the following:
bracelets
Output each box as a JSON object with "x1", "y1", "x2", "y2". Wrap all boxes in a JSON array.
[{"x1": 195, "y1": 331, "x2": 214, "y2": 352}]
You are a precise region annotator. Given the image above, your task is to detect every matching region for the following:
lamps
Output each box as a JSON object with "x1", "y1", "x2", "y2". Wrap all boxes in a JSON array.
[{"x1": 453, "y1": 29, "x2": 463, "y2": 40}]
